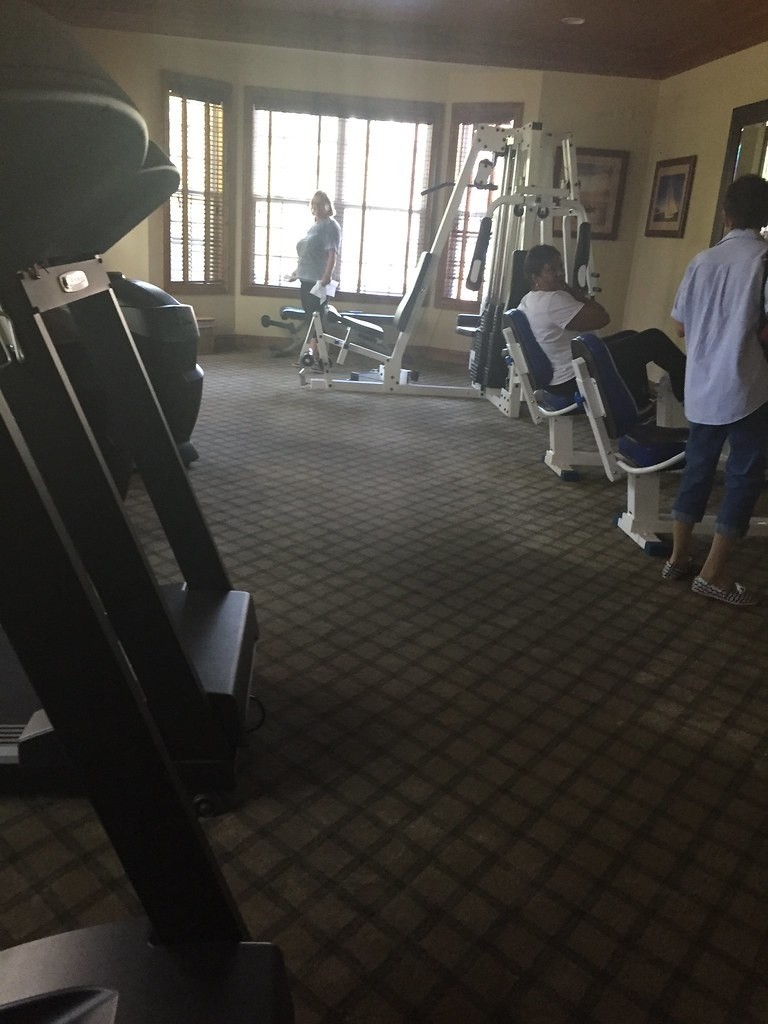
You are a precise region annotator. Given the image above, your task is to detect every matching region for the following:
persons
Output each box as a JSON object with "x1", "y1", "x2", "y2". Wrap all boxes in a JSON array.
[
  {"x1": 662, "y1": 173, "x2": 768, "y2": 604},
  {"x1": 289, "y1": 190, "x2": 341, "y2": 372},
  {"x1": 517, "y1": 245, "x2": 686, "y2": 426}
]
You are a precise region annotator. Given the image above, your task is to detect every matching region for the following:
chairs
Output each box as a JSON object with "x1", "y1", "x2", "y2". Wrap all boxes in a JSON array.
[
  {"x1": 324, "y1": 251, "x2": 432, "y2": 341},
  {"x1": 570, "y1": 331, "x2": 768, "y2": 557},
  {"x1": 502, "y1": 308, "x2": 730, "y2": 482}
]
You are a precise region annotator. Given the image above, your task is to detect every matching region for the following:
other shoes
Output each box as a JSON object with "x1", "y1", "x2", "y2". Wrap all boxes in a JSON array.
[
  {"x1": 638, "y1": 397, "x2": 658, "y2": 425},
  {"x1": 692, "y1": 574, "x2": 759, "y2": 605},
  {"x1": 662, "y1": 555, "x2": 693, "y2": 581},
  {"x1": 310, "y1": 363, "x2": 323, "y2": 373}
]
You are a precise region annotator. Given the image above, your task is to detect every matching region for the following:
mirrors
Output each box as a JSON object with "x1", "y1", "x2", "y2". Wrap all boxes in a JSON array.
[{"x1": 707, "y1": 99, "x2": 768, "y2": 248}]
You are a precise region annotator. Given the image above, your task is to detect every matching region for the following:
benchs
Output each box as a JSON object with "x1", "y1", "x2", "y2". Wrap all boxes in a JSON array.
[{"x1": 261, "y1": 304, "x2": 421, "y2": 373}]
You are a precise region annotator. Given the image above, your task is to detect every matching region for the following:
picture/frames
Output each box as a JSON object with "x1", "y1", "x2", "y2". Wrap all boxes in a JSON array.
[
  {"x1": 645, "y1": 155, "x2": 697, "y2": 238},
  {"x1": 551, "y1": 147, "x2": 630, "y2": 241}
]
